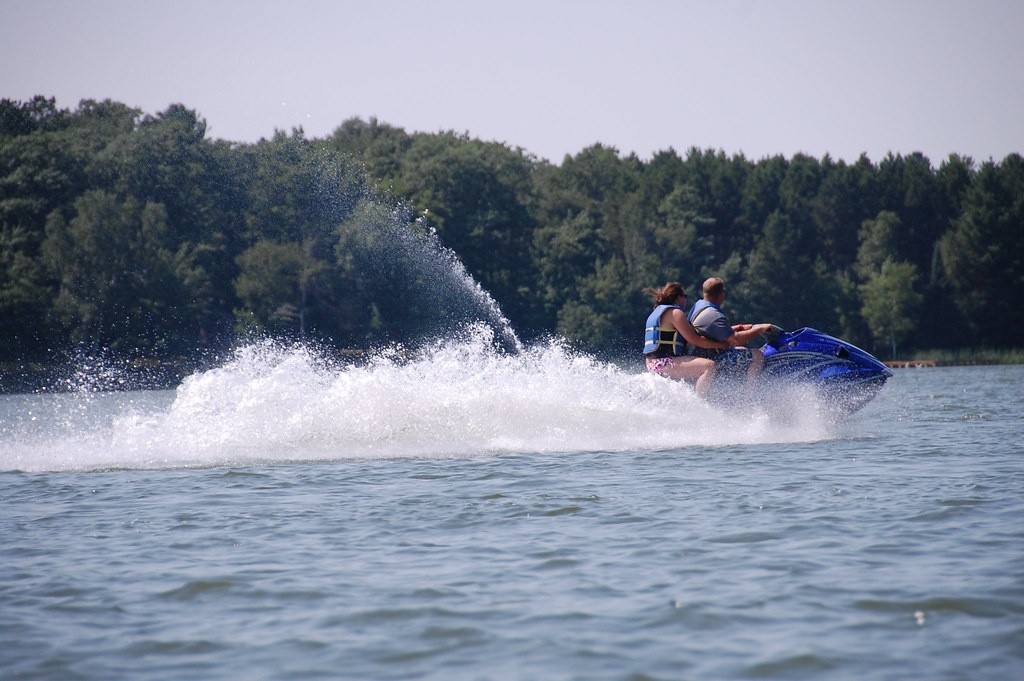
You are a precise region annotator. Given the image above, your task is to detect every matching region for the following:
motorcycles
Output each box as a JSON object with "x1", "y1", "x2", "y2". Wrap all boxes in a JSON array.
[{"x1": 649, "y1": 324, "x2": 895, "y2": 419}]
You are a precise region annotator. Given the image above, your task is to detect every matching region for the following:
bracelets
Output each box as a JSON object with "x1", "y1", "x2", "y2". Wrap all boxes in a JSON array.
[{"x1": 736, "y1": 324, "x2": 744, "y2": 332}]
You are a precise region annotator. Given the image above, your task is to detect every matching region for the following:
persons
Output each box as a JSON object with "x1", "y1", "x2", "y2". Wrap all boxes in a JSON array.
[
  {"x1": 643, "y1": 282, "x2": 732, "y2": 403},
  {"x1": 685, "y1": 276, "x2": 774, "y2": 405}
]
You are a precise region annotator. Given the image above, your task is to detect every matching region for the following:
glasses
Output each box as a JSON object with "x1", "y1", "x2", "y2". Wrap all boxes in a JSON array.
[{"x1": 678, "y1": 293, "x2": 688, "y2": 298}]
